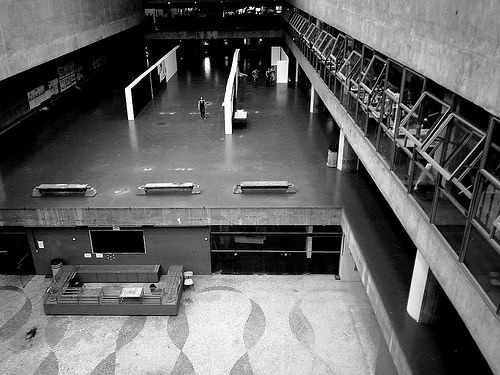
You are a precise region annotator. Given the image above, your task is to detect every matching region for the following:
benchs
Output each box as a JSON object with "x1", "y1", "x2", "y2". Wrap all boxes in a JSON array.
[
  {"x1": 32, "y1": 183, "x2": 97, "y2": 198},
  {"x1": 137, "y1": 183, "x2": 200, "y2": 194},
  {"x1": 232, "y1": 181, "x2": 296, "y2": 194}
]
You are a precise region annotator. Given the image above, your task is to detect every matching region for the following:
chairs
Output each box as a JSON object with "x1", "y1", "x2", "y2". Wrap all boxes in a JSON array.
[{"x1": 183, "y1": 271, "x2": 194, "y2": 292}]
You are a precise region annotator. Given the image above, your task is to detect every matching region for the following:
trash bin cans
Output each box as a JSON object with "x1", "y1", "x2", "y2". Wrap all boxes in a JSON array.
[
  {"x1": 51, "y1": 258, "x2": 62, "y2": 279},
  {"x1": 326, "y1": 143, "x2": 339, "y2": 167}
]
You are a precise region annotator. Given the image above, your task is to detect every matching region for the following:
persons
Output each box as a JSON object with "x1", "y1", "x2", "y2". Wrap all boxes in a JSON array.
[
  {"x1": 198, "y1": 96, "x2": 206, "y2": 119},
  {"x1": 252, "y1": 68, "x2": 259, "y2": 87},
  {"x1": 270, "y1": 66, "x2": 276, "y2": 85},
  {"x1": 266, "y1": 68, "x2": 270, "y2": 85}
]
